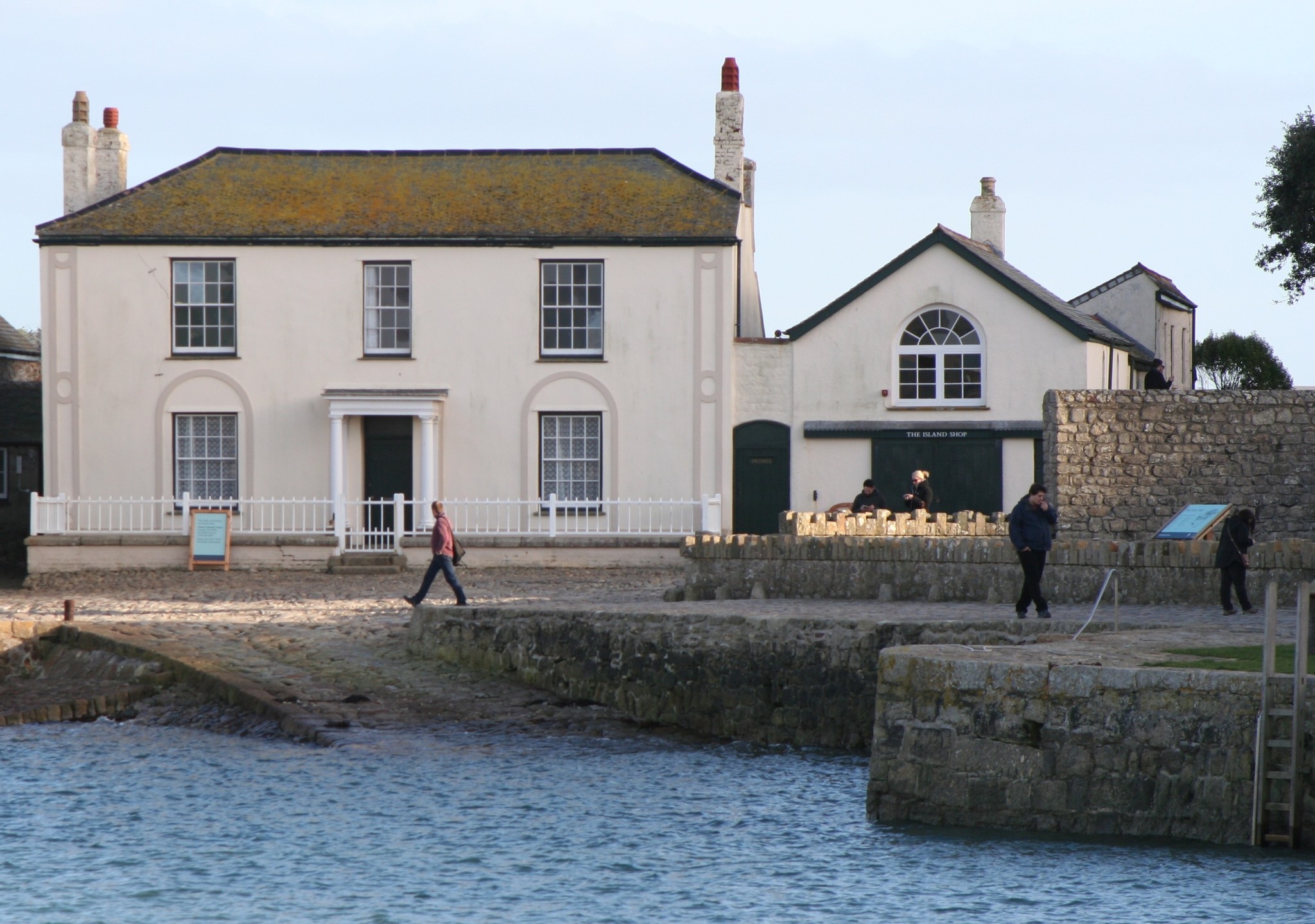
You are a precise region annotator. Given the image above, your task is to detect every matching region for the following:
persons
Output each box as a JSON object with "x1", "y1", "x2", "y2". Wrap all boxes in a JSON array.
[
  {"x1": 1144, "y1": 358, "x2": 1174, "y2": 390},
  {"x1": 403, "y1": 500, "x2": 467, "y2": 606},
  {"x1": 1008, "y1": 483, "x2": 1057, "y2": 618},
  {"x1": 851, "y1": 479, "x2": 892, "y2": 513},
  {"x1": 1214, "y1": 509, "x2": 1260, "y2": 615},
  {"x1": 903, "y1": 470, "x2": 933, "y2": 523}
]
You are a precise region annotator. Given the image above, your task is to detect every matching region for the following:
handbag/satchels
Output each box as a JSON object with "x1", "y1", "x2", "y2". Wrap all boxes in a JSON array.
[
  {"x1": 452, "y1": 533, "x2": 465, "y2": 566},
  {"x1": 1241, "y1": 554, "x2": 1250, "y2": 569}
]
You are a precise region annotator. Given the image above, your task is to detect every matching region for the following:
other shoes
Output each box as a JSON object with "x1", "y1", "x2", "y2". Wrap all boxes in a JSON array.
[
  {"x1": 1016, "y1": 612, "x2": 1027, "y2": 619},
  {"x1": 454, "y1": 600, "x2": 466, "y2": 607},
  {"x1": 404, "y1": 595, "x2": 418, "y2": 608},
  {"x1": 1223, "y1": 609, "x2": 1238, "y2": 616},
  {"x1": 1037, "y1": 610, "x2": 1051, "y2": 618},
  {"x1": 1244, "y1": 607, "x2": 1258, "y2": 614}
]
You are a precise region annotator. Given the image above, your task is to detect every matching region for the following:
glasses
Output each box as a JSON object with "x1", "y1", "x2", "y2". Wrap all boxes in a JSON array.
[{"x1": 912, "y1": 479, "x2": 921, "y2": 482}]
